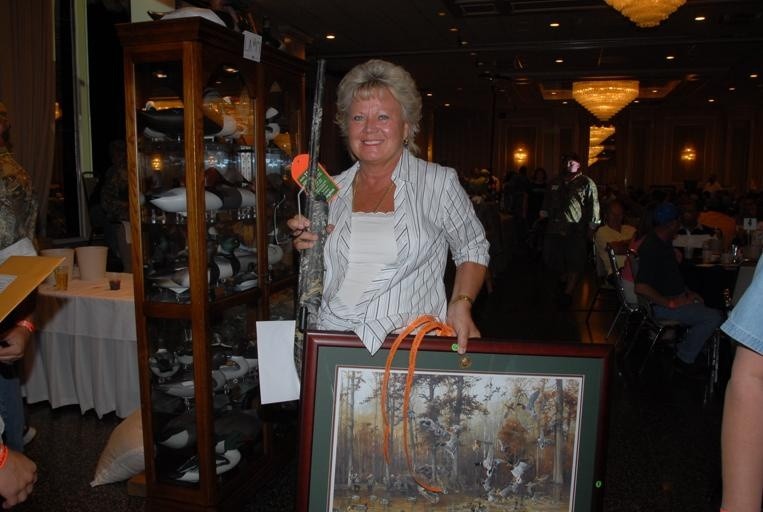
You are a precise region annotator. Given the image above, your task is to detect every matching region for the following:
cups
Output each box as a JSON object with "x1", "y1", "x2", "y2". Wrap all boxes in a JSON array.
[
  {"x1": 680, "y1": 235, "x2": 696, "y2": 259},
  {"x1": 108, "y1": 272, "x2": 122, "y2": 290},
  {"x1": 53, "y1": 265, "x2": 71, "y2": 292},
  {"x1": 701, "y1": 249, "x2": 711, "y2": 263},
  {"x1": 719, "y1": 253, "x2": 729, "y2": 263}
]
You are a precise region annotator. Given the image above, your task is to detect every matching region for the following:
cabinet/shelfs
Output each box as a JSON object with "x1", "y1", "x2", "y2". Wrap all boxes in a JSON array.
[{"x1": 119, "y1": 17, "x2": 319, "y2": 511}]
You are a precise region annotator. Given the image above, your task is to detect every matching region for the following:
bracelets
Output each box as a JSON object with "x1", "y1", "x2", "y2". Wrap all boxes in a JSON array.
[{"x1": 446, "y1": 295, "x2": 476, "y2": 306}]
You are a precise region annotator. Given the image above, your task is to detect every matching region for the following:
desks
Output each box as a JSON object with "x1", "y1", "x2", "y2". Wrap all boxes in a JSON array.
[{"x1": 20, "y1": 271, "x2": 142, "y2": 418}]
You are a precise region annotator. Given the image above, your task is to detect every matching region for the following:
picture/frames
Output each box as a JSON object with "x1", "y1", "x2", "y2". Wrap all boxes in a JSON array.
[{"x1": 297, "y1": 330, "x2": 613, "y2": 512}]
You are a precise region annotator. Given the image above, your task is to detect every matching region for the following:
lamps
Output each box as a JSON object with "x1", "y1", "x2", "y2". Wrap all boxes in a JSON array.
[
  {"x1": 606, "y1": 0, "x2": 691, "y2": 29},
  {"x1": 571, "y1": 79, "x2": 641, "y2": 166}
]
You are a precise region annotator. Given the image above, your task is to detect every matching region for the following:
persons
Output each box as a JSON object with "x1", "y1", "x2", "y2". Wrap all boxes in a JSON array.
[
  {"x1": 287, "y1": 58, "x2": 491, "y2": 356},
  {"x1": 0, "y1": 105, "x2": 39, "y2": 512},
  {"x1": 720, "y1": 246, "x2": 763, "y2": 512},
  {"x1": 470, "y1": 157, "x2": 762, "y2": 375}
]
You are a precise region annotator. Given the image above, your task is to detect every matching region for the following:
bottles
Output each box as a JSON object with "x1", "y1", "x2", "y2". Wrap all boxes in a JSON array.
[{"x1": 730, "y1": 239, "x2": 742, "y2": 263}]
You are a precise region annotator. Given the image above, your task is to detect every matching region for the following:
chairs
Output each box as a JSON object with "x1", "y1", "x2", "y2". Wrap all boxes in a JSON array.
[
  {"x1": 584, "y1": 236, "x2": 618, "y2": 323},
  {"x1": 604, "y1": 242, "x2": 653, "y2": 347},
  {"x1": 618, "y1": 246, "x2": 721, "y2": 406}
]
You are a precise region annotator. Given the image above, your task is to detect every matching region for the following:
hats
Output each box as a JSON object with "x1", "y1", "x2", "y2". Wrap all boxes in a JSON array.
[
  {"x1": 652, "y1": 202, "x2": 683, "y2": 225},
  {"x1": 562, "y1": 151, "x2": 581, "y2": 161}
]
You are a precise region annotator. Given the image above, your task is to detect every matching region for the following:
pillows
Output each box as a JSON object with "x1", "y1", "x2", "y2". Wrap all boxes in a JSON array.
[{"x1": 90, "y1": 410, "x2": 145, "y2": 488}]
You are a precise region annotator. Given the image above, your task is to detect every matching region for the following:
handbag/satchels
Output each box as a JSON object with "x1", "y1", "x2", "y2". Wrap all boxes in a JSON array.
[{"x1": 528, "y1": 216, "x2": 555, "y2": 249}]
